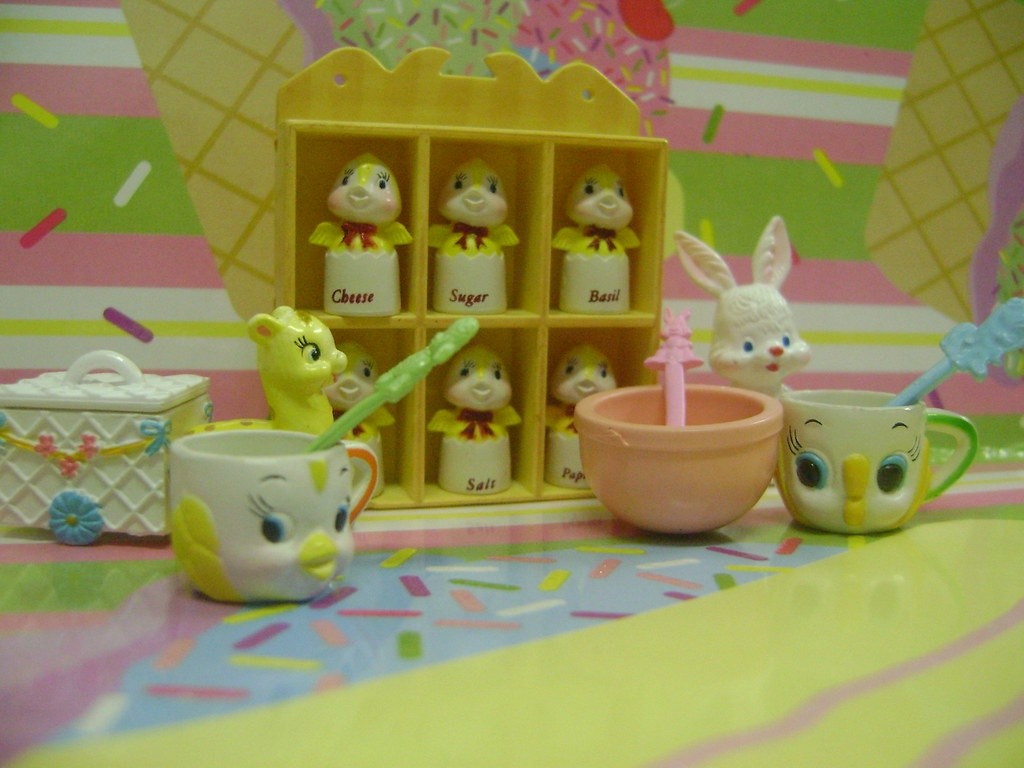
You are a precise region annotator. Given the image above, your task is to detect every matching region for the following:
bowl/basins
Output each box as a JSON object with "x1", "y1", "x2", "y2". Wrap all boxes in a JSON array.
[{"x1": 575, "y1": 382, "x2": 783, "y2": 532}]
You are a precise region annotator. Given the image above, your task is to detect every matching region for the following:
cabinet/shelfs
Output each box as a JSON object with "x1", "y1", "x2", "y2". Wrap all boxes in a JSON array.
[{"x1": 275, "y1": 46, "x2": 671, "y2": 510}]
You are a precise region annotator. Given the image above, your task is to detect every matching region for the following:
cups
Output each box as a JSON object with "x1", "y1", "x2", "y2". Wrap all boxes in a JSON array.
[
  {"x1": 171, "y1": 428, "x2": 379, "y2": 600},
  {"x1": 776, "y1": 389, "x2": 979, "y2": 534}
]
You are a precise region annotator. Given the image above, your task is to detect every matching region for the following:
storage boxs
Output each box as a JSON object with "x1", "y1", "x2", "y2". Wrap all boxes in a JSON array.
[{"x1": 0, "y1": 350, "x2": 212, "y2": 546}]
